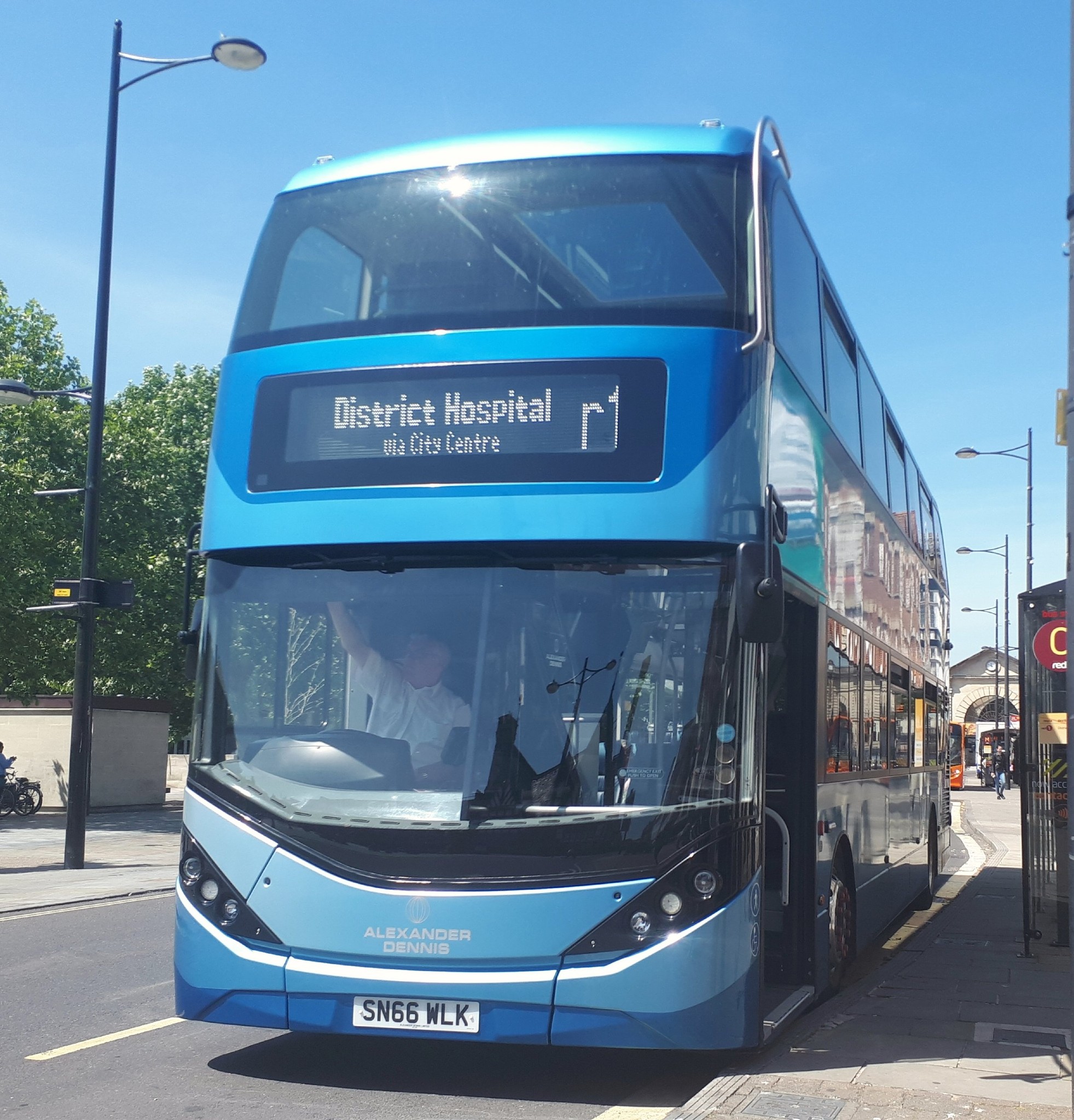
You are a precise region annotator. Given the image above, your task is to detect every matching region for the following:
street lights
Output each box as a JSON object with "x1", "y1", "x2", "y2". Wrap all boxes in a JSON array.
[
  {"x1": 961, "y1": 599, "x2": 999, "y2": 749},
  {"x1": 1, "y1": 19, "x2": 270, "y2": 868},
  {"x1": 954, "y1": 427, "x2": 1035, "y2": 590},
  {"x1": 955, "y1": 533, "x2": 1011, "y2": 791}
]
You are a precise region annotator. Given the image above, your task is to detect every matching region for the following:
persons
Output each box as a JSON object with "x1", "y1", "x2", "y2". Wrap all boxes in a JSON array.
[
  {"x1": 991, "y1": 745, "x2": 1008, "y2": 800},
  {"x1": 328, "y1": 600, "x2": 472, "y2": 783},
  {"x1": 0, "y1": 742, "x2": 17, "y2": 794}
]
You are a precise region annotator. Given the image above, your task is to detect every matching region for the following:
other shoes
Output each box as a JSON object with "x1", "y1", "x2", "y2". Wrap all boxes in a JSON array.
[
  {"x1": 999, "y1": 793, "x2": 1005, "y2": 799},
  {"x1": 997, "y1": 796, "x2": 1001, "y2": 800}
]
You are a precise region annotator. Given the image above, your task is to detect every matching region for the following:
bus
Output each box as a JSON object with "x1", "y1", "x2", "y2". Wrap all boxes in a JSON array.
[
  {"x1": 950, "y1": 721, "x2": 968, "y2": 790},
  {"x1": 825, "y1": 715, "x2": 897, "y2": 773},
  {"x1": 168, "y1": 116, "x2": 953, "y2": 1057}
]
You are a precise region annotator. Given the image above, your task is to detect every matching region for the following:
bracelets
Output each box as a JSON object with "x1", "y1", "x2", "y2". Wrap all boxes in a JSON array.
[{"x1": 422, "y1": 773, "x2": 428, "y2": 780}]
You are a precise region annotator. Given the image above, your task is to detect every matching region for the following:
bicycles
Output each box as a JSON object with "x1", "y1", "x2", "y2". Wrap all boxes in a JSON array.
[{"x1": 0, "y1": 766, "x2": 43, "y2": 818}]
